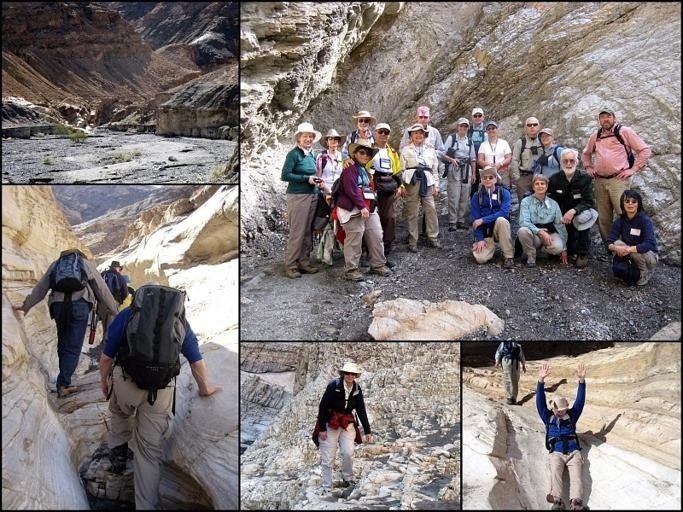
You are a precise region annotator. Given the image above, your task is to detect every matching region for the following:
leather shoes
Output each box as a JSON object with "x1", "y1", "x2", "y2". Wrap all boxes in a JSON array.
[{"x1": 58, "y1": 385, "x2": 78, "y2": 399}]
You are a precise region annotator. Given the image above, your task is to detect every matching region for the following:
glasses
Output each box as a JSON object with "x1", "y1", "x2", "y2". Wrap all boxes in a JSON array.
[
  {"x1": 624, "y1": 199, "x2": 636, "y2": 203},
  {"x1": 482, "y1": 176, "x2": 493, "y2": 180},
  {"x1": 418, "y1": 116, "x2": 428, "y2": 119},
  {"x1": 527, "y1": 123, "x2": 537, "y2": 127},
  {"x1": 562, "y1": 159, "x2": 575, "y2": 164}
]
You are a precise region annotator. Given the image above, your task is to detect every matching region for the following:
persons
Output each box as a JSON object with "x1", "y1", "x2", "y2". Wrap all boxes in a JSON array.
[
  {"x1": 99, "y1": 260, "x2": 128, "y2": 331},
  {"x1": 535, "y1": 362, "x2": 589, "y2": 512},
  {"x1": 99, "y1": 281, "x2": 222, "y2": 510},
  {"x1": 494, "y1": 341, "x2": 526, "y2": 404},
  {"x1": 12, "y1": 248, "x2": 118, "y2": 399},
  {"x1": 279, "y1": 105, "x2": 659, "y2": 288},
  {"x1": 311, "y1": 362, "x2": 372, "y2": 495}
]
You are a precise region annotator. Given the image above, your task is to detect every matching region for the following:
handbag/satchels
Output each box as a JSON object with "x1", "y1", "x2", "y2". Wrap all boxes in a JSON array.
[
  {"x1": 374, "y1": 175, "x2": 397, "y2": 195},
  {"x1": 612, "y1": 254, "x2": 640, "y2": 286},
  {"x1": 442, "y1": 147, "x2": 455, "y2": 178},
  {"x1": 313, "y1": 191, "x2": 330, "y2": 231}
]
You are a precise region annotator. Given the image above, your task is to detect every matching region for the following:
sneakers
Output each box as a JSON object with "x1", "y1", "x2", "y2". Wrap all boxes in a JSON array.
[
  {"x1": 636, "y1": 270, "x2": 654, "y2": 285},
  {"x1": 550, "y1": 497, "x2": 565, "y2": 510},
  {"x1": 596, "y1": 254, "x2": 613, "y2": 262},
  {"x1": 286, "y1": 269, "x2": 302, "y2": 278},
  {"x1": 569, "y1": 498, "x2": 589, "y2": 511},
  {"x1": 430, "y1": 241, "x2": 439, "y2": 247},
  {"x1": 448, "y1": 223, "x2": 456, "y2": 231},
  {"x1": 457, "y1": 222, "x2": 469, "y2": 229},
  {"x1": 344, "y1": 256, "x2": 396, "y2": 281},
  {"x1": 299, "y1": 267, "x2": 319, "y2": 274},
  {"x1": 503, "y1": 254, "x2": 588, "y2": 269},
  {"x1": 108, "y1": 454, "x2": 128, "y2": 474},
  {"x1": 407, "y1": 244, "x2": 419, "y2": 252}
]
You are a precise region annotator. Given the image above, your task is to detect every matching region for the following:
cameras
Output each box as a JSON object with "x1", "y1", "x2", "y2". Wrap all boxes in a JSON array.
[{"x1": 460, "y1": 160, "x2": 469, "y2": 184}]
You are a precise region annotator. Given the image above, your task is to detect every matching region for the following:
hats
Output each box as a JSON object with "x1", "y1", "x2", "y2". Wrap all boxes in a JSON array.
[
  {"x1": 457, "y1": 107, "x2": 497, "y2": 131},
  {"x1": 109, "y1": 261, "x2": 123, "y2": 267},
  {"x1": 549, "y1": 398, "x2": 569, "y2": 411},
  {"x1": 61, "y1": 247, "x2": 87, "y2": 259},
  {"x1": 572, "y1": 207, "x2": 598, "y2": 231},
  {"x1": 537, "y1": 128, "x2": 552, "y2": 139},
  {"x1": 598, "y1": 108, "x2": 613, "y2": 116},
  {"x1": 294, "y1": 111, "x2": 391, "y2": 160},
  {"x1": 338, "y1": 362, "x2": 361, "y2": 378},
  {"x1": 478, "y1": 166, "x2": 502, "y2": 180},
  {"x1": 123, "y1": 275, "x2": 131, "y2": 283},
  {"x1": 407, "y1": 123, "x2": 429, "y2": 137}
]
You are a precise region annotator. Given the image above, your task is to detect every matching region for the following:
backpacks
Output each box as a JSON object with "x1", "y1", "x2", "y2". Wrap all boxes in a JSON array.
[
  {"x1": 103, "y1": 272, "x2": 123, "y2": 304},
  {"x1": 117, "y1": 285, "x2": 188, "y2": 416},
  {"x1": 501, "y1": 341, "x2": 520, "y2": 371},
  {"x1": 597, "y1": 124, "x2": 635, "y2": 169},
  {"x1": 50, "y1": 253, "x2": 89, "y2": 293}
]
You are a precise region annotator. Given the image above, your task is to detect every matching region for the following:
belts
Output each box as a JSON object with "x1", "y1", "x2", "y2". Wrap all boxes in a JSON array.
[{"x1": 596, "y1": 174, "x2": 616, "y2": 179}]
